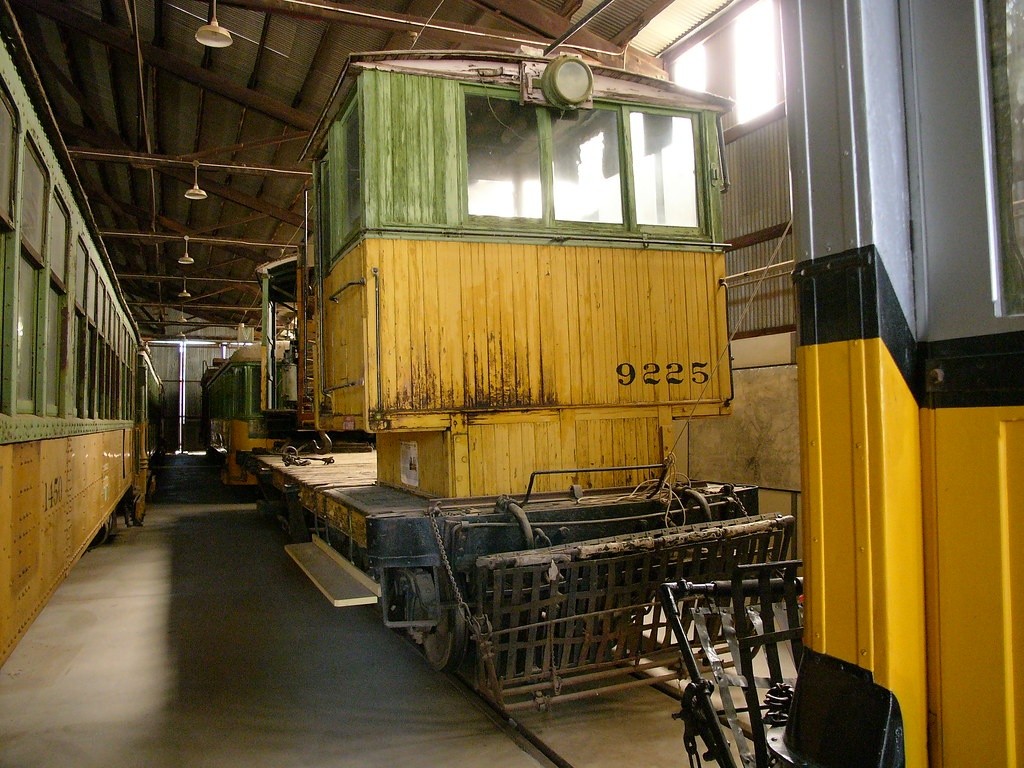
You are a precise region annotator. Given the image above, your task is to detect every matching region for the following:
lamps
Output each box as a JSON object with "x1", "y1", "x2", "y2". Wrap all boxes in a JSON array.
[
  {"x1": 178, "y1": 235, "x2": 195, "y2": 264},
  {"x1": 176, "y1": 323, "x2": 186, "y2": 336},
  {"x1": 194, "y1": 1, "x2": 233, "y2": 47},
  {"x1": 177, "y1": 304, "x2": 187, "y2": 322},
  {"x1": 184, "y1": 160, "x2": 208, "y2": 199},
  {"x1": 178, "y1": 276, "x2": 191, "y2": 297}
]
